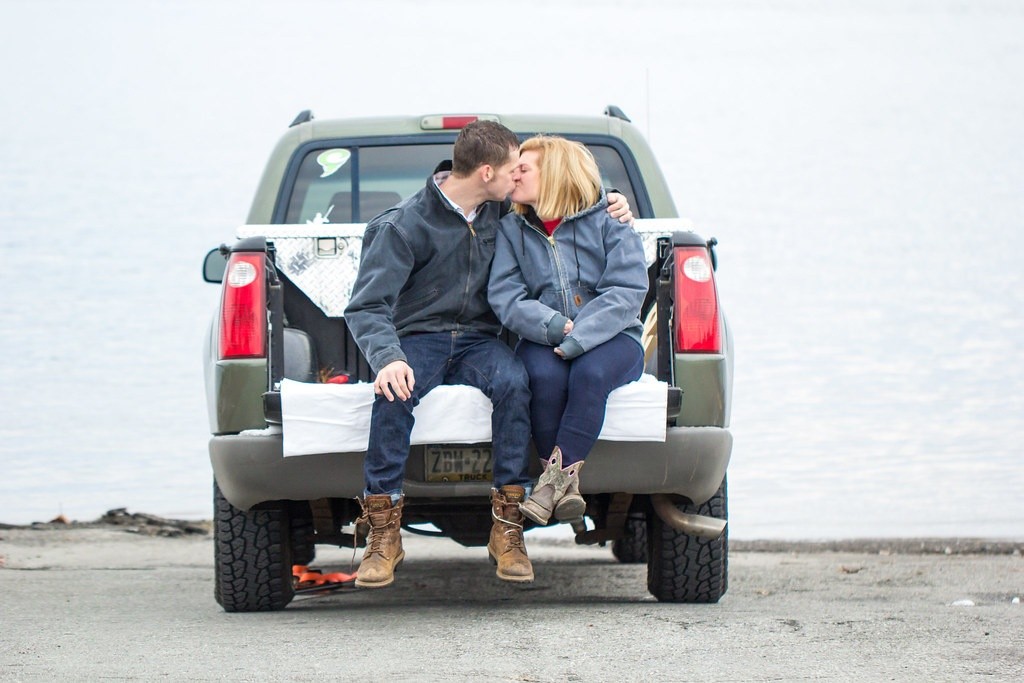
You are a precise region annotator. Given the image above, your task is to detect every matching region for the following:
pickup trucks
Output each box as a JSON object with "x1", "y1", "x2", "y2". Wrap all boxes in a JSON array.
[{"x1": 201, "y1": 104, "x2": 733, "y2": 615}]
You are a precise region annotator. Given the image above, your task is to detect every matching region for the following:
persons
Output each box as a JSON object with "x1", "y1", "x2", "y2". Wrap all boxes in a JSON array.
[
  {"x1": 487, "y1": 134, "x2": 649, "y2": 525},
  {"x1": 344, "y1": 121, "x2": 634, "y2": 589}
]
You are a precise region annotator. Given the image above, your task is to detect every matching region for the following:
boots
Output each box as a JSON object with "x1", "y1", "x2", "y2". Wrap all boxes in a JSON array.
[
  {"x1": 487, "y1": 486, "x2": 535, "y2": 586},
  {"x1": 520, "y1": 445, "x2": 584, "y2": 526},
  {"x1": 537, "y1": 459, "x2": 590, "y2": 535},
  {"x1": 352, "y1": 497, "x2": 405, "y2": 590}
]
